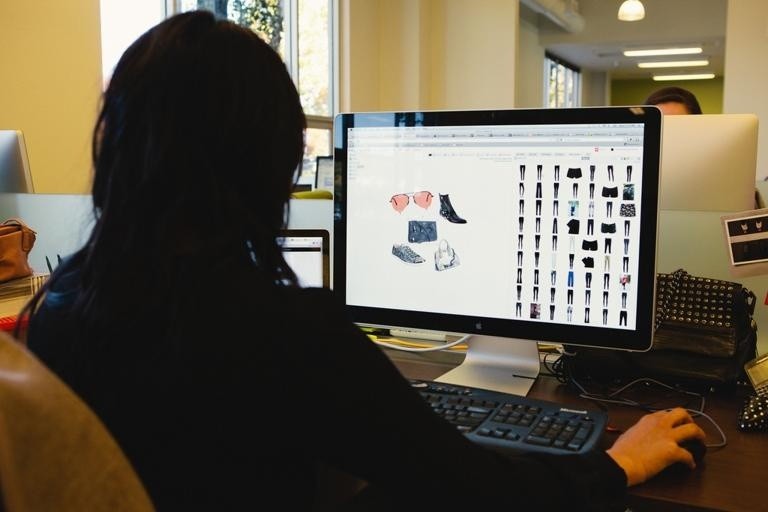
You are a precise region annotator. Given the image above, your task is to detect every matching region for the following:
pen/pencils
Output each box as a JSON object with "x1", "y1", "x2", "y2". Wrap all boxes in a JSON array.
[
  {"x1": 381, "y1": 329, "x2": 446, "y2": 342},
  {"x1": 46, "y1": 256, "x2": 52, "y2": 274},
  {"x1": 57, "y1": 255, "x2": 61, "y2": 264}
]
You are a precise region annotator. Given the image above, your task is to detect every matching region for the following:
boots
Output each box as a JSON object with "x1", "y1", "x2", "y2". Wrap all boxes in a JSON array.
[{"x1": 439, "y1": 192, "x2": 467, "y2": 224}]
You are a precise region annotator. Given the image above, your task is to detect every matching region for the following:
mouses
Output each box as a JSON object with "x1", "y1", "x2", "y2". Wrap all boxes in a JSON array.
[{"x1": 652, "y1": 437, "x2": 707, "y2": 476}]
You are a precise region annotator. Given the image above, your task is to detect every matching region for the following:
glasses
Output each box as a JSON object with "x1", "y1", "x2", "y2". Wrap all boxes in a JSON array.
[{"x1": 389, "y1": 191, "x2": 434, "y2": 215}]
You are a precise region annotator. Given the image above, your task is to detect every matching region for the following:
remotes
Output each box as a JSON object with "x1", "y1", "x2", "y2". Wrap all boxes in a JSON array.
[{"x1": 275, "y1": 228, "x2": 330, "y2": 289}]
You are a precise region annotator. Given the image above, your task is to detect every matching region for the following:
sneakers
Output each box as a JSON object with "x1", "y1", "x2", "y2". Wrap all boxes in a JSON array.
[{"x1": 392, "y1": 243, "x2": 425, "y2": 264}]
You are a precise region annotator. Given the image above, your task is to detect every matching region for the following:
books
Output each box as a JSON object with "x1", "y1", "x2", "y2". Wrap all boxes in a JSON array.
[{"x1": 0, "y1": 274, "x2": 49, "y2": 299}]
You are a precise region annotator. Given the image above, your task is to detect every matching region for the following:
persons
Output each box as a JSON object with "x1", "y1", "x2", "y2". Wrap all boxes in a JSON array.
[
  {"x1": 25, "y1": 8, "x2": 708, "y2": 512},
  {"x1": 644, "y1": 87, "x2": 703, "y2": 115}
]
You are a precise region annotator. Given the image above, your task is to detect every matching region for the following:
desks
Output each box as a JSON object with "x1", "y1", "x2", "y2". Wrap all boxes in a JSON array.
[{"x1": 356, "y1": 322, "x2": 767, "y2": 511}]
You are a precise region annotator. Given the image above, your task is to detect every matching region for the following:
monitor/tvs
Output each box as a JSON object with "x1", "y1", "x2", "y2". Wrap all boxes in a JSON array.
[
  {"x1": 659, "y1": 114, "x2": 758, "y2": 211},
  {"x1": 315, "y1": 155, "x2": 333, "y2": 191},
  {"x1": 0, "y1": 129, "x2": 35, "y2": 193},
  {"x1": 333, "y1": 105, "x2": 664, "y2": 398}
]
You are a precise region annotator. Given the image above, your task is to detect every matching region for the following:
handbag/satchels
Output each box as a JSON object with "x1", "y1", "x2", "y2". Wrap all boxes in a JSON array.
[
  {"x1": 435, "y1": 239, "x2": 460, "y2": 270},
  {"x1": 562, "y1": 269, "x2": 757, "y2": 401},
  {"x1": 408, "y1": 220, "x2": 437, "y2": 242},
  {"x1": 0, "y1": 218, "x2": 36, "y2": 283}
]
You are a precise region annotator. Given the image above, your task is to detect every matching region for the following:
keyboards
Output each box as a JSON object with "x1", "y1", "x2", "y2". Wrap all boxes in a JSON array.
[{"x1": 408, "y1": 379, "x2": 610, "y2": 455}]
